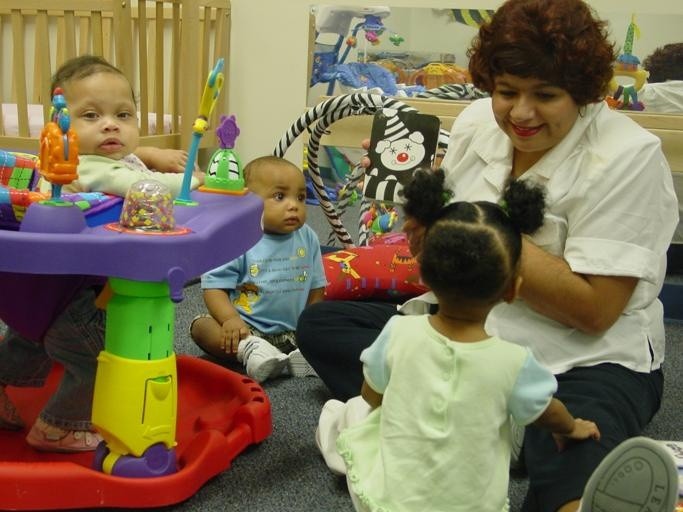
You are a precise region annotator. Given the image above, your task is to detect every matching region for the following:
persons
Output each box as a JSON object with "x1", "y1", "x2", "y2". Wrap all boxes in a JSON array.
[
  {"x1": 295, "y1": 0, "x2": 681, "y2": 512},
  {"x1": 190, "y1": 156, "x2": 328, "y2": 385},
  {"x1": 0, "y1": 55, "x2": 190, "y2": 454},
  {"x1": 315, "y1": 166, "x2": 602, "y2": 512}
]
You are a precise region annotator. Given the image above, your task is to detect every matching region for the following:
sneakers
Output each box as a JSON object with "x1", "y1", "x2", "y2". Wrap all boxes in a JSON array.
[
  {"x1": 578, "y1": 437, "x2": 680, "y2": 512},
  {"x1": 237, "y1": 334, "x2": 319, "y2": 383},
  {"x1": 25, "y1": 419, "x2": 103, "y2": 452},
  {"x1": 1, "y1": 389, "x2": 25, "y2": 430}
]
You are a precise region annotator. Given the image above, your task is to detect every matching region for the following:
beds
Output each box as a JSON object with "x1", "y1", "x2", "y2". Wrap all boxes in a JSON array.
[{"x1": 0, "y1": 0, "x2": 230, "y2": 171}]
